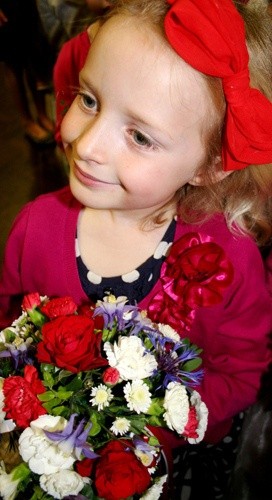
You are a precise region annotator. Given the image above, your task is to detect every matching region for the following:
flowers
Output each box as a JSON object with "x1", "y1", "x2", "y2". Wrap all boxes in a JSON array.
[
  {"x1": 0, "y1": 292, "x2": 208, "y2": 500},
  {"x1": 148, "y1": 230, "x2": 238, "y2": 333}
]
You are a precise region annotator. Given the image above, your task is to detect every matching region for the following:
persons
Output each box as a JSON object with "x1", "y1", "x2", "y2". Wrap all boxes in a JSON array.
[{"x1": 0, "y1": 0, "x2": 272, "y2": 500}]
